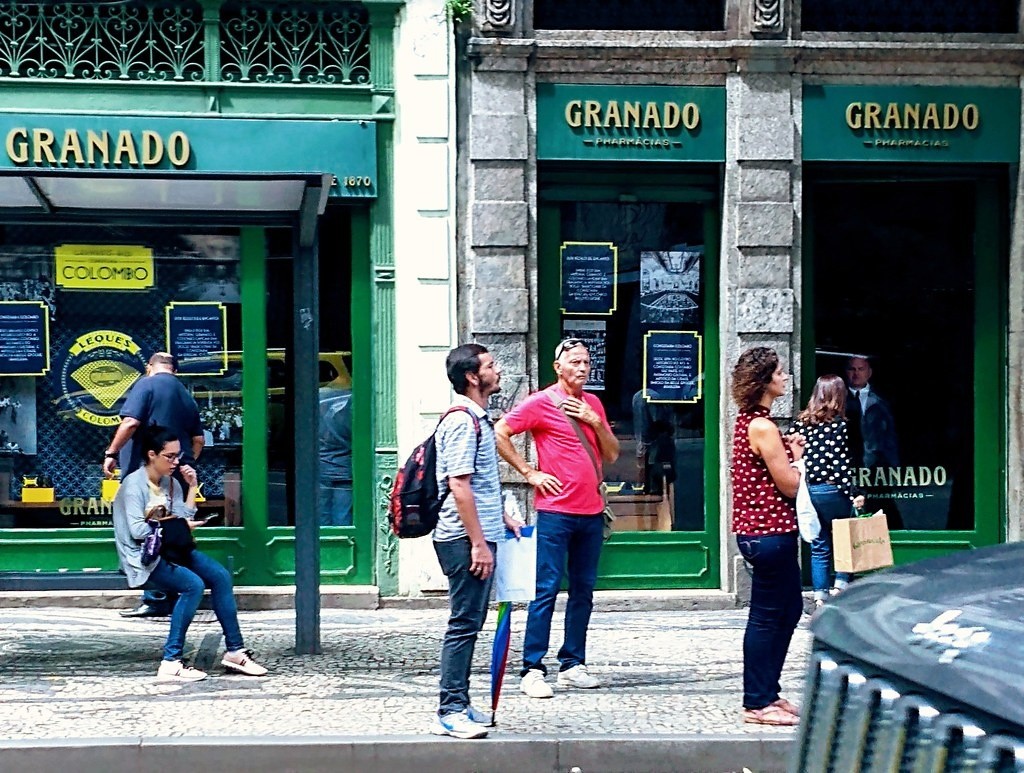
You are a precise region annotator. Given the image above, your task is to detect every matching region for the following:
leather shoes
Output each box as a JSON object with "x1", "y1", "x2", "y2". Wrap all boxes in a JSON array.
[{"x1": 119, "y1": 603, "x2": 167, "y2": 617}]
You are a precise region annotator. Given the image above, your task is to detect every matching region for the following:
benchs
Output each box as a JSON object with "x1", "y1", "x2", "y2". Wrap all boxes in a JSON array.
[{"x1": 0, "y1": 570, "x2": 187, "y2": 590}]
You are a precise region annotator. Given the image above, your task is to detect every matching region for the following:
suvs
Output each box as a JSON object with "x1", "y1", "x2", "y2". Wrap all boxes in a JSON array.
[{"x1": 785, "y1": 542, "x2": 1024, "y2": 773}]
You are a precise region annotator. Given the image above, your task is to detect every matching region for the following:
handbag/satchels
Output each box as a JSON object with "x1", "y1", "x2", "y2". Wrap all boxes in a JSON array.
[
  {"x1": 831, "y1": 506, "x2": 893, "y2": 573},
  {"x1": 599, "y1": 482, "x2": 615, "y2": 545},
  {"x1": 145, "y1": 505, "x2": 178, "y2": 524},
  {"x1": 792, "y1": 460, "x2": 821, "y2": 543}
]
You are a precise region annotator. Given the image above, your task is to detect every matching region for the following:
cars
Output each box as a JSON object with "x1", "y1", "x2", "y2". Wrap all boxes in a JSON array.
[{"x1": 54, "y1": 350, "x2": 351, "y2": 415}]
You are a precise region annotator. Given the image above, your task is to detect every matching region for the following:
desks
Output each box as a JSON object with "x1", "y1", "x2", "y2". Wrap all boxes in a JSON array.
[{"x1": 0, "y1": 472, "x2": 242, "y2": 526}]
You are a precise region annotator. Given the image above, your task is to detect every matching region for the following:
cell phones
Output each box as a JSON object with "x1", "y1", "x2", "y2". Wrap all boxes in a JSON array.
[{"x1": 193, "y1": 513, "x2": 219, "y2": 521}]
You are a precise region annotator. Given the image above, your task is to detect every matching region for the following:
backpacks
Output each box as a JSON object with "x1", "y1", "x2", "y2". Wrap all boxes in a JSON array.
[{"x1": 388, "y1": 407, "x2": 481, "y2": 538}]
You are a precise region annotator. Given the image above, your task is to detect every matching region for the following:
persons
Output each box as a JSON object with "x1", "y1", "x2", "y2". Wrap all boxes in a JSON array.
[
  {"x1": 317, "y1": 359, "x2": 353, "y2": 526},
  {"x1": 846, "y1": 354, "x2": 899, "y2": 471},
  {"x1": 113, "y1": 426, "x2": 269, "y2": 682},
  {"x1": 432, "y1": 343, "x2": 524, "y2": 740},
  {"x1": 785, "y1": 375, "x2": 865, "y2": 608},
  {"x1": 730, "y1": 347, "x2": 807, "y2": 726},
  {"x1": 493, "y1": 338, "x2": 620, "y2": 698},
  {"x1": 632, "y1": 382, "x2": 679, "y2": 530},
  {"x1": 102, "y1": 352, "x2": 205, "y2": 617}
]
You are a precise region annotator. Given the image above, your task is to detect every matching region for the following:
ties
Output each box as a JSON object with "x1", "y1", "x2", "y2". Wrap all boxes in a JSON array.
[{"x1": 855, "y1": 390, "x2": 862, "y2": 422}]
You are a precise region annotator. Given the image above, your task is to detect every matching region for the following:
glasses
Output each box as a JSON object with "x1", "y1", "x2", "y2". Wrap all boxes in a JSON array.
[
  {"x1": 158, "y1": 451, "x2": 184, "y2": 463},
  {"x1": 557, "y1": 339, "x2": 589, "y2": 360}
]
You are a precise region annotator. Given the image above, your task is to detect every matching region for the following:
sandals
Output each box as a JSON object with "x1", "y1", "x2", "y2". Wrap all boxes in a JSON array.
[
  {"x1": 745, "y1": 704, "x2": 800, "y2": 725},
  {"x1": 775, "y1": 699, "x2": 800, "y2": 717}
]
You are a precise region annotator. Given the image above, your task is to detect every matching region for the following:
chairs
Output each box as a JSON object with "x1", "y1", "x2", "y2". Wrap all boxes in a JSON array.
[{"x1": 599, "y1": 449, "x2": 676, "y2": 530}]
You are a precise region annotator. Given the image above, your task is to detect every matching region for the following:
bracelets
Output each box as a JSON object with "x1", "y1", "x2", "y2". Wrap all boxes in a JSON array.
[{"x1": 104, "y1": 450, "x2": 119, "y2": 461}]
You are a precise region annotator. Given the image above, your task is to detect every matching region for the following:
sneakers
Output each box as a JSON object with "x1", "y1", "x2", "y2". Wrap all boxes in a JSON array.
[
  {"x1": 157, "y1": 658, "x2": 208, "y2": 682},
  {"x1": 430, "y1": 708, "x2": 488, "y2": 739},
  {"x1": 221, "y1": 647, "x2": 268, "y2": 675},
  {"x1": 468, "y1": 705, "x2": 498, "y2": 726},
  {"x1": 520, "y1": 667, "x2": 553, "y2": 697},
  {"x1": 557, "y1": 664, "x2": 599, "y2": 688}
]
